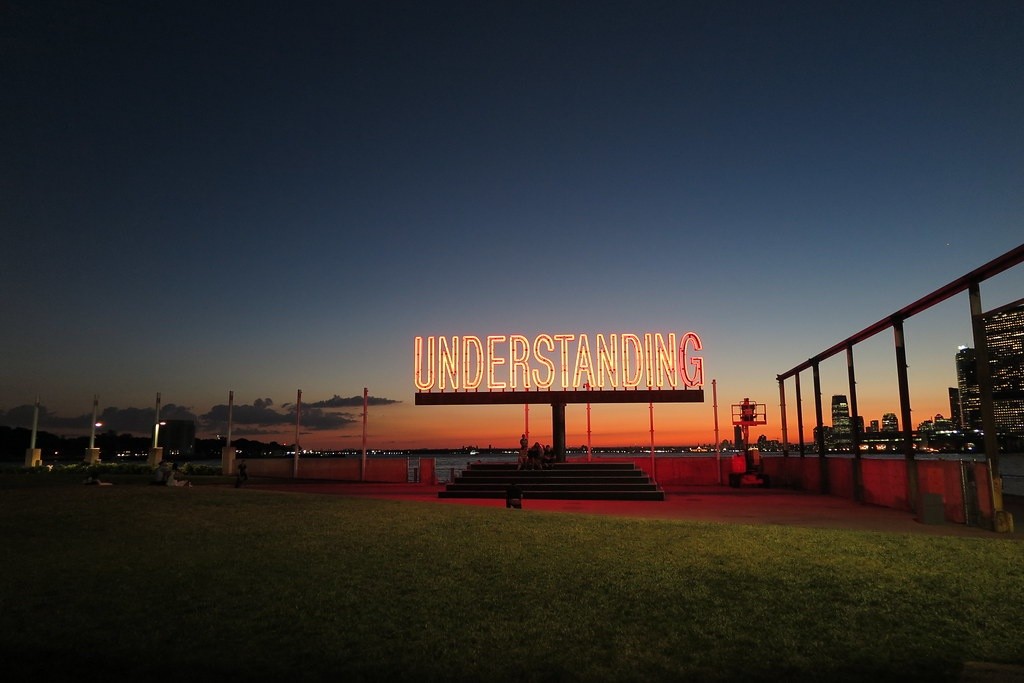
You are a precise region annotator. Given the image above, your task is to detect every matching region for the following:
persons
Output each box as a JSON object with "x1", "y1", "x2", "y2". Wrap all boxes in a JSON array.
[
  {"x1": 152, "y1": 461, "x2": 193, "y2": 488},
  {"x1": 505, "y1": 482, "x2": 523, "y2": 508},
  {"x1": 237, "y1": 460, "x2": 248, "y2": 487},
  {"x1": 517, "y1": 434, "x2": 556, "y2": 470}
]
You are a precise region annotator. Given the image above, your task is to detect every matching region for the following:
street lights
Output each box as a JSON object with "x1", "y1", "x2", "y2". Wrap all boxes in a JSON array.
[
  {"x1": 90, "y1": 399, "x2": 103, "y2": 448},
  {"x1": 154, "y1": 392, "x2": 166, "y2": 447}
]
